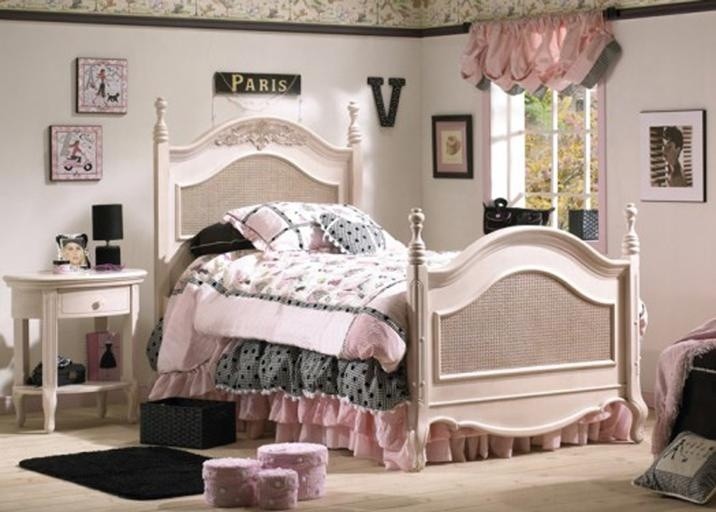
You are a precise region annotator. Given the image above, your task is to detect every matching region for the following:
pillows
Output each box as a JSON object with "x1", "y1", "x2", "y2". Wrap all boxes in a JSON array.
[
  {"x1": 223, "y1": 202, "x2": 405, "y2": 256},
  {"x1": 632, "y1": 431, "x2": 715, "y2": 506},
  {"x1": 188, "y1": 220, "x2": 254, "y2": 255},
  {"x1": 317, "y1": 212, "x2": 385, "y2": 255}
]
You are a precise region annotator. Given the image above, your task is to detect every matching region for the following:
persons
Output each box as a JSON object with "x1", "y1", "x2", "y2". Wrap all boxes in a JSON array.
[
  {"x1": 661, "y1": 126, "x2": 688, "y2": 187},
  {"x1": 68, "y1": 140, "x2": 87, "y2": 164},
  {"x1": 61, "y1": 240, "x2": 83, "y2": 269},
  {"x1": 91, "y1": 69, "x2": 110, "y2": 105}
]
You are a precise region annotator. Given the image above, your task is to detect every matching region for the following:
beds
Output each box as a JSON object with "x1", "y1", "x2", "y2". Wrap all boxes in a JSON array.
[{"x1": 150, "y1": 97, "x2": 639, "y2": 467}]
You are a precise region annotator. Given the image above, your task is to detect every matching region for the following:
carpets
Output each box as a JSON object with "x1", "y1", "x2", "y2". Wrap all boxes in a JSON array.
[{"x1": 19, "y1": 449, "x2": 219, "y2": 499}]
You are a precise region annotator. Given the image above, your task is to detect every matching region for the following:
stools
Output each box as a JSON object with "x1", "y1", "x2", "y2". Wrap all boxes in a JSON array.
[{"x1": 661, "y1": 318, "x2": 716, "y2": 446}]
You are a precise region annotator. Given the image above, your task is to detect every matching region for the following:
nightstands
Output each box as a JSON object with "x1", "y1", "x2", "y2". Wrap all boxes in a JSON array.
[{"x1": 4, "y1": 270, "x2": 146, "y2": 431}]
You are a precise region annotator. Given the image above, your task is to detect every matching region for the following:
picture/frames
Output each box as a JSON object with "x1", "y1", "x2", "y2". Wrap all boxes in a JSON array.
[
  {"x1": 432, "y1": 115, "x2": 473, "y2": 180},
  {"x1": 49, "y1": 125, "x2": 103, "y2": 181},
  {"x1": 639, "y1": 109, "x2": 707, "y2": 203},
  {"x1": 76, "y1": 57, "x2": 128, "y2": 114}
]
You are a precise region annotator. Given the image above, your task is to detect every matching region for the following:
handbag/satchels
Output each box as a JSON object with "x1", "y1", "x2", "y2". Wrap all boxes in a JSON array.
[{"x1": 483, "y1": 199, "x2": 554, "y2": 233}]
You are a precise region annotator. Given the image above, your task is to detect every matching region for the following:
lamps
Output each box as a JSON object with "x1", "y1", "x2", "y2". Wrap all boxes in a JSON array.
[{"x1": 92, "y1": 204, "x2": 122, "y2": 270}]
